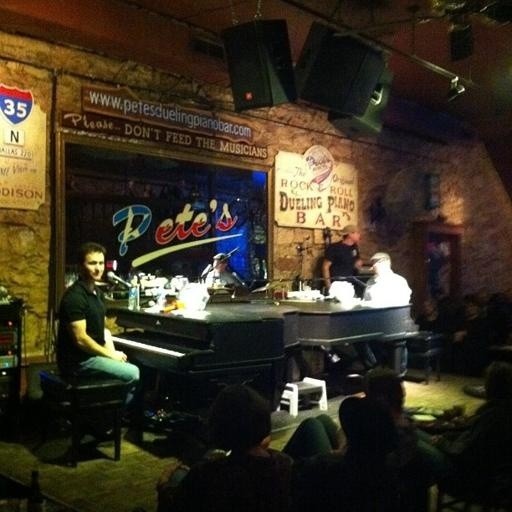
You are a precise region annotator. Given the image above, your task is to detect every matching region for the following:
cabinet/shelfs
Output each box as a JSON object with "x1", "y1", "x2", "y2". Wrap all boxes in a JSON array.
[{"x1": 1, "y1": 297, "x2": 24, "y2": 415}]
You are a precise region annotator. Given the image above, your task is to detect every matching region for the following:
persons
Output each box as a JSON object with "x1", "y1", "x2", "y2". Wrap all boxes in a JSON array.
[
  {"x1": 56, "y1": 241, "x2": 143, "y2": 423},
  {"x1": 280, "y1": 366, "x2": 419, "y2": 511},
  {"x1": 158, "y1": 381, "x2": 295, "y2": 510},
  {"x1": 409, "y1": 294, "x2": 511, "y2": 346},
  {"x1": 206, "y1": 253, "x2": 242, "y2": 286},
  {"x1": 320, "y1": 225, "x2": 367, "y2": 298},
  {"x1": 363, "y1": 252, "x2": 413, "y2": 305},
  {"x1": 420, "y1": 359, "x2": 510, "y2": 505},
  {"x1": 166, "y1": 415, "x2": 212, "y2": 485}
]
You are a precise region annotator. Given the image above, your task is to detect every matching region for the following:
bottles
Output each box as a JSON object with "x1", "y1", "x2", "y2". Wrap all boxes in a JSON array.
[{"x1": 128, "y1": 285, "x2": 139, "y2": 311}]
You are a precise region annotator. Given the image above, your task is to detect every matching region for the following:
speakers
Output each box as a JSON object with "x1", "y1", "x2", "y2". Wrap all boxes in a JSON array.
[
  {"x1": 221, "y1": 18, "x2": 298, "y2": 113},
  {"x1": 294, "y1": 19, "x2": 388, "y2": 117},
  {"x1": 328, "y1": 65, "x2": 395, "y2": 139}
]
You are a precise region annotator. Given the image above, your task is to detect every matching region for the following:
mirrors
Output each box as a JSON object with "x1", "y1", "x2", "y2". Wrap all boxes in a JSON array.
[{"x1": 53, "y1": 127, "x2": 275, "y2": 312}]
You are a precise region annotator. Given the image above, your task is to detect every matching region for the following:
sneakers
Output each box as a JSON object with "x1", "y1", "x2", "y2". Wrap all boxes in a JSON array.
[{"x1": 463, "y1": 385, "x2": 487, "y2": 398}]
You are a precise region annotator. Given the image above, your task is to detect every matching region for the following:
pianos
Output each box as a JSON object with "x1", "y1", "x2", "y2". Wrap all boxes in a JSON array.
[
  {"x1": 296, "y1": 302, "x2": 412, "y2": 396},
  {"x1": 106, "y1": 306, "x2": 297, "y2": 437}
]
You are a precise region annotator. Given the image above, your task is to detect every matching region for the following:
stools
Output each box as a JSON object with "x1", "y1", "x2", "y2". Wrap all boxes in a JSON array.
[
  {"x1": 407, "y1": 330, "x2": 445, "y2": 384},
  {"x1": 276, "y1": 376, "x2": 329, "y2": 419},
  {"x1": 41, "y1": 370, "x2": 132, "y2": 467}
]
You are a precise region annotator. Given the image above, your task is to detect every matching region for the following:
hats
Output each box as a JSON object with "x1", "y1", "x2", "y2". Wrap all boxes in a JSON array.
[
  {"x1": 213, "y1": 253, "x2": 226, "y2": 260},
  {"x1": 340, "y1": 225, "x2": 358, "y2": 235},
  {"x1": 371, "y1": 253, "x2": 391, "y2": 261}
]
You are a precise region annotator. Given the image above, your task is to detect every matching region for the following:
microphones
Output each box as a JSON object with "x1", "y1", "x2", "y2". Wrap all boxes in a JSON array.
[
  {"x1": 200, "y1": 263, "x2": 212, "y2": 278},
  {"x1": 106, "y1": 271, "x2": 132, "y2": 287},
  {"x1": 232, "y1": 271, "x2": 246, "y2": 287}
]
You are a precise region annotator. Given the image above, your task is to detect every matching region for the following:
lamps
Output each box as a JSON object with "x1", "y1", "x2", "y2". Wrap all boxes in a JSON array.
[{"x1": 443, "y1": 75, "x2": 469, "y2": 101}]
